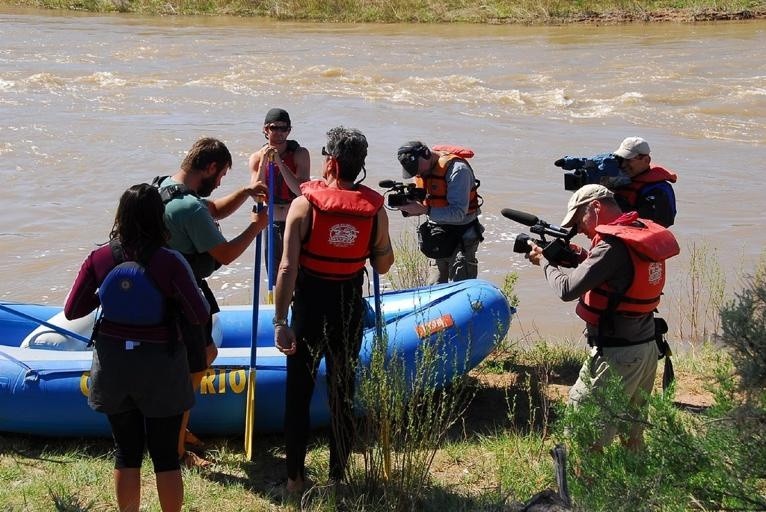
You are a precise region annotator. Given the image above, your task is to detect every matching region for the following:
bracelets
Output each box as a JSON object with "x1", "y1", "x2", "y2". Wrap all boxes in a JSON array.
[{"x1": 271, "y1": 315, "x2": 288, "y2": 326}]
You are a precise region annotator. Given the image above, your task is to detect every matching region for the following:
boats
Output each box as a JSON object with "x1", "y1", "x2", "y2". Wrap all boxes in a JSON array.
[{"x1": 0, "y1": 276, "x2": 515, "y2": 437}]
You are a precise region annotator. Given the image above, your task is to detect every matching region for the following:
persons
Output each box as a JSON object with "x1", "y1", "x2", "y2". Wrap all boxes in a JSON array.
[
  {"x1": 522, "y1": 184, "x2": 681, "y2": 478},
  {"x1": 606, "y1": 135, "x2": 677, "y2": 230},
  {"x1": 249, "y1": 108, "x2": 310, "y2": 301},
  {"x1": 148, "y1": 137, "x2": 269, "y2": 470},
  {"x1": 62, "y1": 182, "x2": 209, "y2": 512},
  {"x1": 270, "y1": 126, "x2": 394, "y2": 498},
  {"x1": 391, "y1": 141, "x2": 485, "y2": 282}
]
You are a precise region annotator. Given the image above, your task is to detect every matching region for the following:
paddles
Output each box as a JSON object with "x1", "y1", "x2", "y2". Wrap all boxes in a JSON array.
[
  {"x1": 244, "y1": 206, "x2": 262, "y2": 463},
  {"x1": 269, "y1": 152, "x2": 274, "y2": 305}
]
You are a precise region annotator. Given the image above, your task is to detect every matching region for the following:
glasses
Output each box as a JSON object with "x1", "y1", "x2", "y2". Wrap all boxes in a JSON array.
[{"x1": 268, "y1": 125, "x2": 289, "y2": 132}]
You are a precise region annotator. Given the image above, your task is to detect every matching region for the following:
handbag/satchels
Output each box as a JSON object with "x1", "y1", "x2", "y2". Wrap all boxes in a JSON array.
[{"x1": 419, "y1": 222, "x2": 459, "y2": 259}]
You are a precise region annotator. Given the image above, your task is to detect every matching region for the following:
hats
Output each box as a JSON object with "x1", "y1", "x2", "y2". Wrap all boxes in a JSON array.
[
  {"x1": 561, "y1": 184, "x2": 615, "y2": 227},
  {"x1": 613, "y1": 137, "x2": 650, "y2": 159},
  {"x1": 265, "y1": 109, "x2": 289, "y2": 124},
  {"x1": 398, "y1": 142, "x2": 421, "y2": 179}
]
